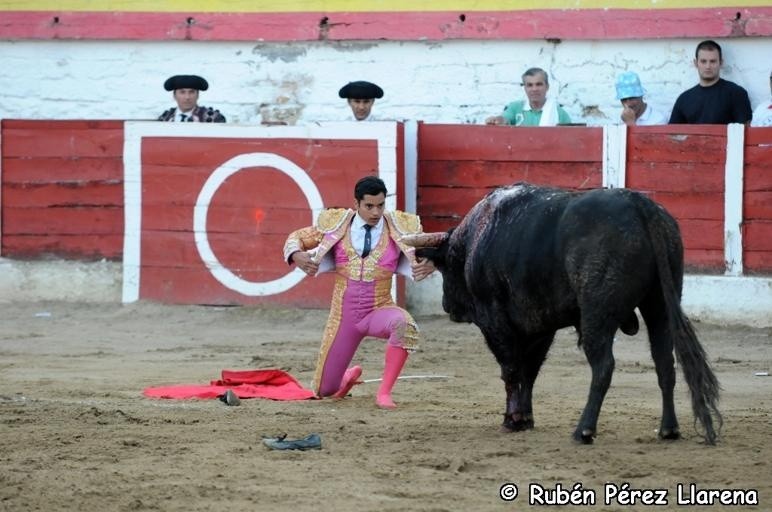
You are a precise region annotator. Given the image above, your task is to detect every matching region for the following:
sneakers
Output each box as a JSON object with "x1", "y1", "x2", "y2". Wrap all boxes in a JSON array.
[
  {"x1": 262, "y1": 434, "x2": 321, "y2": 450},
  {"x1": 220, "y1": 390, "x2": 240, "y2": 406}
]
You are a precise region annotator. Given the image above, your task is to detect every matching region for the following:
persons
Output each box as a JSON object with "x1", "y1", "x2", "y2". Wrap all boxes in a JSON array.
[
  {"x1": 155, "y1": 74, "x2": 227, "y2": 122},
  {"x1": 750, "y1": 69, "x2": 771, "y2": 147},
  {"x1": 336, "y1": 79, "x2": 395, "y2": 121},
  {"x1": 484, "y1": 66, "x2": 574, "y2": 127},
  {"x1": 281, "y1": 175, "x2": 437, "y2": 409},
  {"x1": 669, "y1": 39, "x2": 754, "y2": 127},
  {"x1": 613, "y1": 69, "x2": 668, "y2": 126}
]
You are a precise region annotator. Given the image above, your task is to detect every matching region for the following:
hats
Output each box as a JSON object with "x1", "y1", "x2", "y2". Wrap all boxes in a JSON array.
[
  {"x1": 164, "y1": 76, "x2": 207, "y2": 91},
  {"x1": 339, "y1": 82, "x2": 383, "y2": 99},
  {"x1": 616, "y1": 73, "x2": 644, "y2": 100}
]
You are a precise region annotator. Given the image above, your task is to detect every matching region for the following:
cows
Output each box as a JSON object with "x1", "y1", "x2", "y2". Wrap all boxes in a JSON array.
[{"x1": 396, "y1": 181, "x2": 726, "y2": 447}]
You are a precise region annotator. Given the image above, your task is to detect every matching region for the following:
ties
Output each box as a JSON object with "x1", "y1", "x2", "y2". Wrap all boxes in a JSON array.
[{"x1": 361, "y1": 224, "x2": 372, "y2": 257}]
